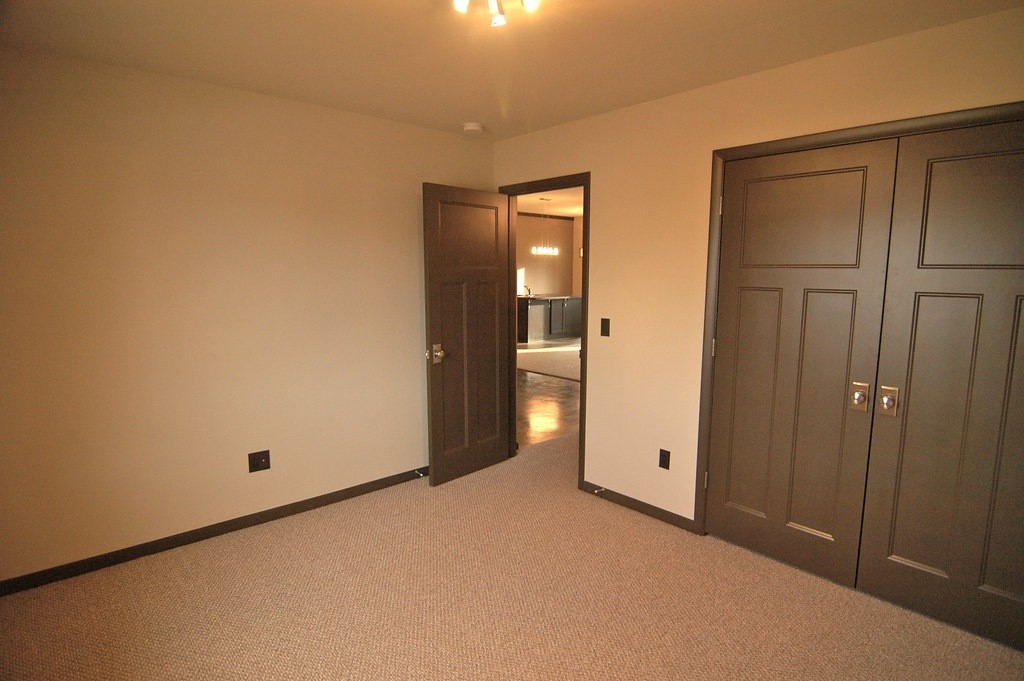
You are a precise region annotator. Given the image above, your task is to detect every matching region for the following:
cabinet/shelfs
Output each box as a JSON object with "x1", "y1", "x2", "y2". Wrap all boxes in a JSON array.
[
  {"x1": 551, "y1": 299, "x2": 581, "y2": 338},
  {"x1": 517, "y1": 297, "x2": 551, "y2": 341}
]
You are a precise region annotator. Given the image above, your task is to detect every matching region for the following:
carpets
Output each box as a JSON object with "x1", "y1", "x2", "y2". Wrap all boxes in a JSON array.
[{"x1": 517, "y1": 345, "x2": 581, "y2": 382}]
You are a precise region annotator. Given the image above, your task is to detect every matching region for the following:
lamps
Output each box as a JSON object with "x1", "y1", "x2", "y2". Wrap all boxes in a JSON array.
[
  {"x1": 453, "y1": 0, "x2": 541, "y2": 27},
  {"x1": 530, "y1": 198, "x2": 559, "y2": 256}
]
